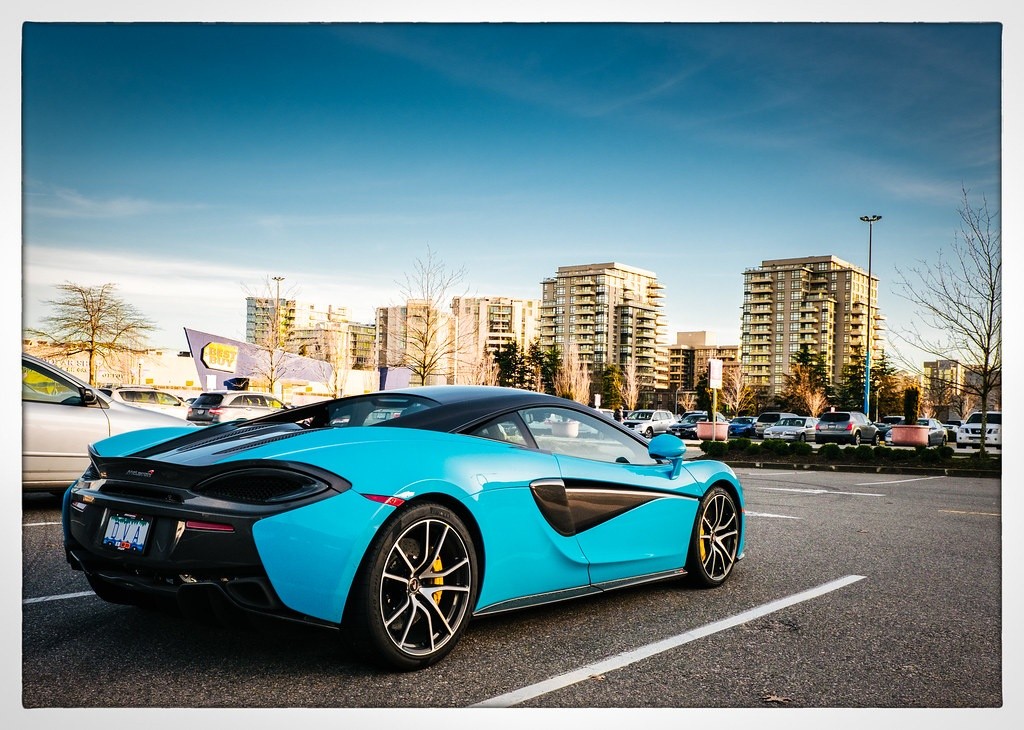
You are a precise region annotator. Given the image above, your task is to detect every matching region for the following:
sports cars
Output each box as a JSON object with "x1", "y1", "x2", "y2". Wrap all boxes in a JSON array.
[{"x1": 56, "y1": 388, "x2": 752, "y2": 669}]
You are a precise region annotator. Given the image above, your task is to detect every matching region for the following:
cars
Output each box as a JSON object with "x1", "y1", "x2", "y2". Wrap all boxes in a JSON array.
[
  {"x1": 557, "y1": 403, "x2": 620, "y2": 439},
  {"x1": 886, "y1": 418, "x2": 949, "y2": 448},
  {"x1": 665, "y1": 413, "x2": 729, "y2": 439},
  {"x1": 677, "y1": 410, "x2": 727, "y2": 423},
  {"x1": 726, "y1": 414, "x2": 759, "y2": 438},
  {"x1": 957, "y1": 410, "x2": 1002, "y2": 451},
  {"x1": 23, "y1": 353, "x2": 199, "y2": 506},
  {"x1": 622, "y1": 407, "x2": 679, "y2": 440},
  {"x1": 764, "y1": 416, "x2": 820, "y2": 444},
  {"x1": 816, "y1": 409, "x2": 882, "y2": 448},
  {"x1": 753, "y1": 412, "x2": 799, "y2": 437}
]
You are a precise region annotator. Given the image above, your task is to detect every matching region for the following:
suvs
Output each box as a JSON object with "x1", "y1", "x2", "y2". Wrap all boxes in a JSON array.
[
  {"x1": 184, "y1": 388, "x2": 291, "y2": 429},
  {"x1": 94, "y1": 385, "x2": 191, "y2": 426}
]
